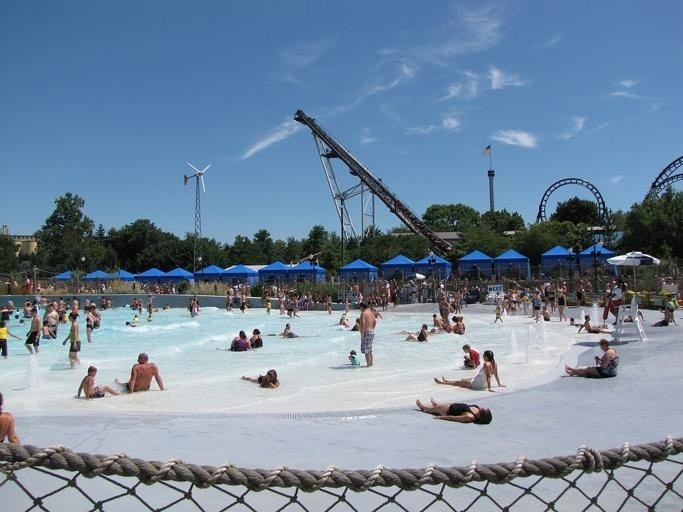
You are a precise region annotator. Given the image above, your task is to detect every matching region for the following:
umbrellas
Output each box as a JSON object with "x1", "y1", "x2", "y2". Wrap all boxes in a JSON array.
[{"x1": 607, "y1": 249, "x2": 661, "y2": 293}]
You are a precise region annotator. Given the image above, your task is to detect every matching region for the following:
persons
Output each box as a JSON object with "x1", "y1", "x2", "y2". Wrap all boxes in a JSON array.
[
  {"x1": 224, "y1": 266, "x2": 401, "y2": 368},
  {"x1": 651, "y1": 277, "x2": 681, "y2": 328},
  {"x1": 1, "y1": 394, "x2": 21, "y2": 444},
  {"x1": 73, "y1": 366, "x2": 125, "y2": 400},
  {"x1": 568, "y1": 275, "x2": 643, "y2": 334},
  {"x1": 397, "y1": 273, "x2": 487, "y2": 344},
  {"x1": 565, "y1": 338, "x2": 619, "y2": 379},
  {"x1": 239, "y1": 369, "x2": 279, "y2": 389},
  {"x1": 187, "y1": 294, "x2": 200, "y2": 318},
  {"x1": 433, "y1": 350, "x2": 506, "y2": 392},
  {"x1": 416, "y1": 396, "x2": 492, "y2": 426},
  {"x1": 114, "y1": 353, "x2": 167, "y2": 394},
  {"x1": 0, "y1": 271, "x2": 183, "y2": 371},
  {"x1": 462, "y1": 344, "x2": 480, "y2": 369},
  {"x1": 491, "y1": 275, "x2": 591, "y2": 324}
]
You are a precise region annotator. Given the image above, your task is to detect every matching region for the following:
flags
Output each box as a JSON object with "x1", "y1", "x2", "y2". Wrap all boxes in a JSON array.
[{"x1": 482, "y1": 144, "x2": 491, "y2": 156}]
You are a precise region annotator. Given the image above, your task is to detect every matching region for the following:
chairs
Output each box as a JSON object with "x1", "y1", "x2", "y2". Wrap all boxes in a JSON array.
[
  {"x1": 485, "y1": 290, "x2": 496, "y2": 303},
  {"x1": 495, "y1": 291, "x2": 506, "y2": 302},
  {"x1": 611, "y1": 295, "x2": 647, "y2": 342}
]
